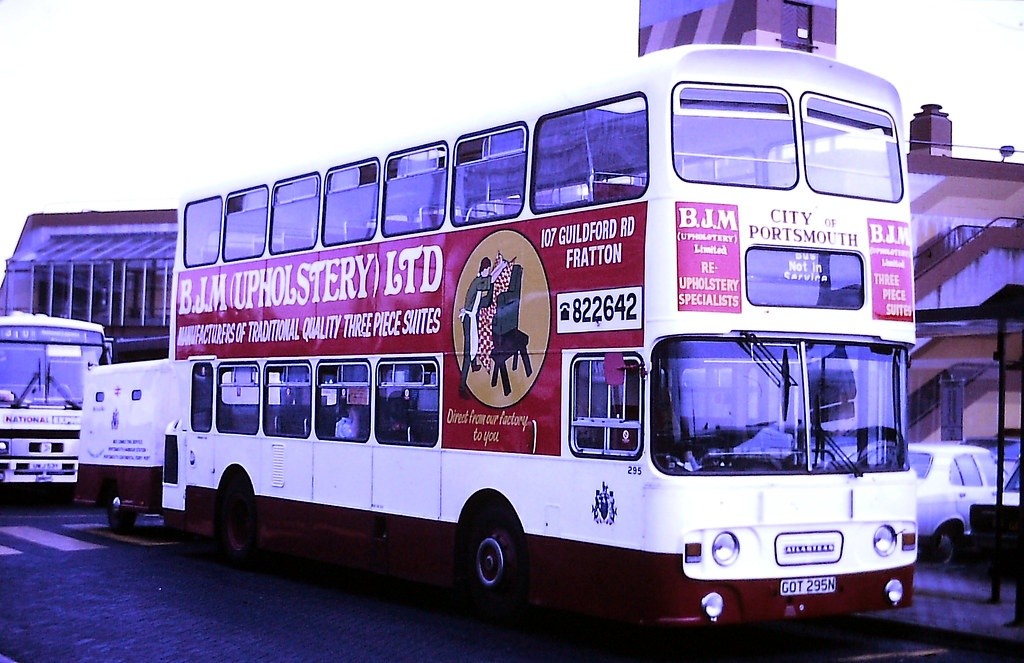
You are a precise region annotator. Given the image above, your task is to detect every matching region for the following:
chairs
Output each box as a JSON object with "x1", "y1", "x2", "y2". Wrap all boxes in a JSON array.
[
  {"x1": 303, "y1": 414, "x2": 336, "y2": 437},
  {"x1": 407, "y1": 422, "x2": 437, "y2": 443},
  {"x1": 273, "y1": 411, "x2": 307, "y2": 435},
  {"x1": 281, "y1": 231, "x2": 313, "y2": 251}
]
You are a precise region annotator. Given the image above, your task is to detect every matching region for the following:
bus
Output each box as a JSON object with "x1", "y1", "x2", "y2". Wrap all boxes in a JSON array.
[
  {"x1": 161, "y1": 41, "x2": 919, "y2": 620},
  {"x1": 0, "y1": 310, "x2": 113, "y2": 504}
]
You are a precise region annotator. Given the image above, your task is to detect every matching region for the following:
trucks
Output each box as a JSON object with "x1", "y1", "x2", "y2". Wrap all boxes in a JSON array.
[{"x1": 73, "y1": 358, "x2": 338, "y2": 536}]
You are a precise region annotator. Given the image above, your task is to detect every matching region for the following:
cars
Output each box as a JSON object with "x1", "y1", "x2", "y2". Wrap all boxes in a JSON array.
[{"x1": 839, "y1": 442, "x2": 1020, "y2": 568}]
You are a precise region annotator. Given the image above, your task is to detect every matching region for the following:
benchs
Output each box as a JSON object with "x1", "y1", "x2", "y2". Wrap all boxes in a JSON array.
[
  {"x1": 310, "y1": 224, "x2": 344, "y2": 246},
  {"x1": 587, "y1": 171, "x2": 646, "y2": 202},
  {"x1": 200, "y1": 244, "x2": 217, "y2": 263},
  {"x1": 252, "y1": 235, "x2": 281, "y2": 255},
  {"x1": 342, "y1": 219, "x2": 375, "y2": 240},
  {"x1": 418, "y1": 206, "x2": 498, "y2": 229},
  {"x1": 463, "y1": 201, "x2": 557, "y2": 222},
  {"x1": 225, "y1": 240, "x2": 253, "y2": 260},
  {"x1": 373, "y1": 213, "x2": 421, "y2": 234}
]
